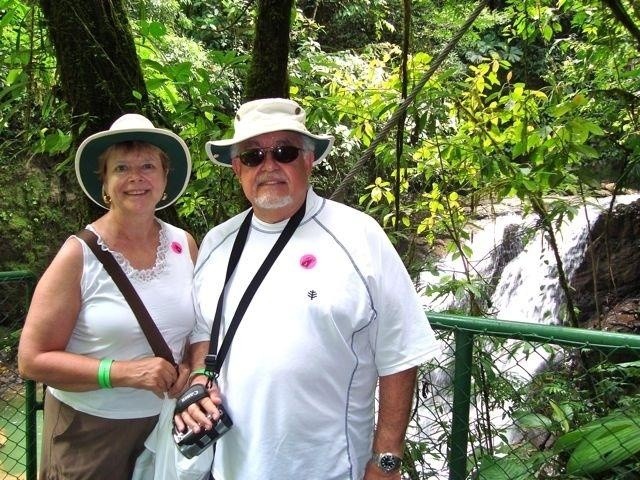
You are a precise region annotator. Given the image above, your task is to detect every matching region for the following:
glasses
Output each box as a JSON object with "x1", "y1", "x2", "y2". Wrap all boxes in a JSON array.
[{"x1": 229, "y1": 145, "x2": 304, "y2": 168}]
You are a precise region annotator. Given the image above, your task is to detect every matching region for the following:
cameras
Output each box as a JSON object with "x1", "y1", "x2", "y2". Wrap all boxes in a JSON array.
[{"x1": 172, "y1": 404, "x2": 233, "y2": 459}]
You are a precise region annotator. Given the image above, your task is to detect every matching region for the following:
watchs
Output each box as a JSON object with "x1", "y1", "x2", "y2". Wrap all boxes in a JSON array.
[{"x1": 372, "y1": 452, "x2": 403, "y2": 473}]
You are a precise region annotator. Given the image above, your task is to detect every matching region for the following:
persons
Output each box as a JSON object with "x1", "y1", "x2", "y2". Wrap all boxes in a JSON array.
[
  {"x1": 173, "y1": 97, "x2": 441, "y2": 480},
  {"x1": 18, "y1": 113, "x2": 199, "y2": 480}
]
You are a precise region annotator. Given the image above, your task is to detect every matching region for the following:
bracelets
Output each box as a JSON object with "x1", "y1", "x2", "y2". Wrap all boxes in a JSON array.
[
  {"x1": 98, "y1": 358, "x2": 114, "y2": 388},
  {"x1": 190, "y1": 368, "x2": 214, "y2": 376}
]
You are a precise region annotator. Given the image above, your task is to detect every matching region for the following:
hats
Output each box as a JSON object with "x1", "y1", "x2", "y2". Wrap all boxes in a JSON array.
[
  {"x1": 74, "y1": 115, "x2": 194, "y2": 212},
  {"x1": 205, "y1": 98, "x2": 335, "y2": 170}
]
